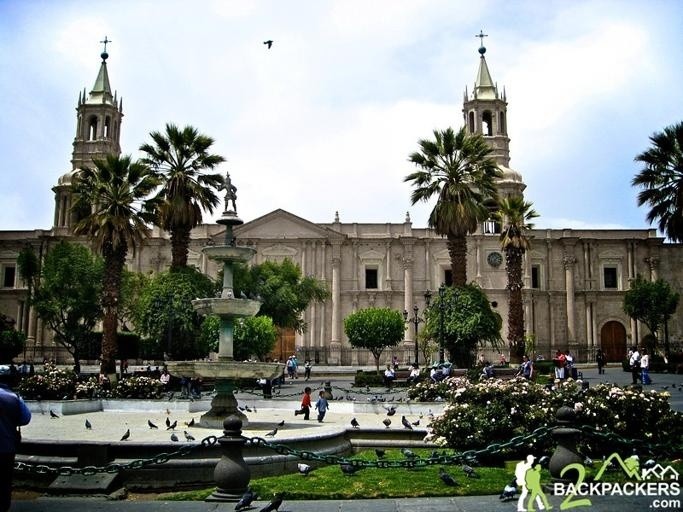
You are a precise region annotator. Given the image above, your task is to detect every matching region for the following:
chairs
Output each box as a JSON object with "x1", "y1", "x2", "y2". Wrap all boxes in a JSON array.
[{"x1": 382, "y1": 369, "x2": 468, "y2": 387}]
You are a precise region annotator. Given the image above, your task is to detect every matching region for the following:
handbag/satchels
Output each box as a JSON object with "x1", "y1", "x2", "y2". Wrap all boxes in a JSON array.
[{"x1": 16, "y1": 430, "x2": 21, "y2": 441}]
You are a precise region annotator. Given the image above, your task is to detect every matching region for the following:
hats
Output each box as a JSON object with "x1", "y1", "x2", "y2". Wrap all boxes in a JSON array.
[
  {"x1": 274, "y1": 359, "x2": 277, "y2": 361},
  {"x1": 293, "y1": 355, "x2": 296, "y2": 358},
  {"x1": 597, "y1": 349, "x2": 601, "y2": 352},
  {"x1": 289, "y1": 356, "x2": 292, "y2": 358}
]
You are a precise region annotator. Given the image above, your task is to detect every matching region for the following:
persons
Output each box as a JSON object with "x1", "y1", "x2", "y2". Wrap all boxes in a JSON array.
[
  {"x1": 4, "y1": 360, "x2": 35, "y2": 384},
  {"x1": 626, "y1": 346, "x2": 633, "y2": 359},
  {"x1": 383, "y1": 348, "x2": 573, "y2": 385},
  {"x1": 0, "y1": 366, "x2": 30, "y2": 510},
  {"x1": 595, "y1": 348, "x2": 604, "y2": 374},
  {"x1": 629, "y1": 346, "x2": 643, "y2": 385},
  {"x1": 216, "y1": 178, "x2": 237, "y2": 213},
  {"x1": 636, "y1": 347, "x2": 652, "y2": 385},
  {"x1": 314, "y1": 390, "x2": 329, "y2": 423},
  {"x1": 270, "y1": 354, "x2": 313, "y2": 379},
  {"x1": 120, "y1": 358, "x2": 203, "y2": 396},
  {"x1": 294, "y1": 386, "x2": 311, "y2": 420}
]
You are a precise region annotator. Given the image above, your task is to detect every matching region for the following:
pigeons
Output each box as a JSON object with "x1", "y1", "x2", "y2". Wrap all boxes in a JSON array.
[
  {"x1": 333, "y1": 393, "x2": 434, "y2": 428},
  {"x1": 233, "y1": 485, "x2": 286, "y2": 512},
  {"x1": 339, "y1": 464, "x2": 359, "y2": 476},
  {"x1": 121, "y1": 429, "x2": 131, "y2": 441},
  {"x1": 297, "y1": 462, "x2": 318, "y2": 476},
  {"x1": 374, "y1": 448, "x2": 442, "y2": 459},
  {"x1": 439, "y1": 459, "x2": 478, "y2": 486},
  {"x1": 499, "y1": 476, "x2": 517, "y2": 500},
  {"x1": 86, "y1": 419, "x2": 92, "y2": 429},
  {"x1": 49, "y1": 410, "x2": 59, "y2": 419},
  {"x1": 146, "y1": 388, "x2": 285, "y2": 441}
]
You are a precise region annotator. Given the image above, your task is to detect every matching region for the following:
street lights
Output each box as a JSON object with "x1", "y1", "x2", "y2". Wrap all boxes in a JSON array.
[
  {"x1": 423, "y1": 289, "x2": 432, "y2": 367},
  {"x1": 438, "y1": 282, "x2": 447, "y2": 370},
  {"x1": 407, "y1": 305, "x2": 425, "y2": 369}
]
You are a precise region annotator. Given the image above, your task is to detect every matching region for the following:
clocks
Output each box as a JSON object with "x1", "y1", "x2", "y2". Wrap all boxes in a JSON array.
[{"x1": 487, "y1": 251, "x2": 503, "y2": 267}]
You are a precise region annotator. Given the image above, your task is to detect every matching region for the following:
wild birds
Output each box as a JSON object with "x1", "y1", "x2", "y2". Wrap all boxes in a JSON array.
[{"x1": 264, "y1": 40, "x2": 273, "y2": 49}]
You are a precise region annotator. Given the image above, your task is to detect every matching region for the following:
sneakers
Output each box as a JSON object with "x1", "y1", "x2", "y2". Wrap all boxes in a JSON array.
[
  {"x1": 386, "y1": 386, "x2": 391, "y2": 391},
  {"x1": 318, "y1": 419, "x2": 323, "y2": 423},
  {"x1": 295, "y1": 410, "x2": 309, "y2": 419},
  {"x1": 631, "y1": 380, "x2": 652, "y2": 385}
]
